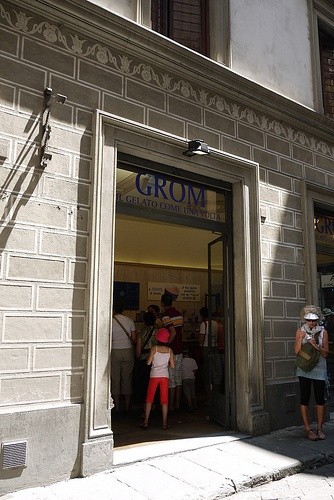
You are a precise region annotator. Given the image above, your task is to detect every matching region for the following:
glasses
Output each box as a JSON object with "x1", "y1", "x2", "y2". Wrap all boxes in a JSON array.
[{"x1": 306, "y1": 319, "x2": 317, "y2": 322}]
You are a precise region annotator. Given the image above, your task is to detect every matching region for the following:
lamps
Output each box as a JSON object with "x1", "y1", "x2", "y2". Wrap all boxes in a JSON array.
[{"x1": 182, "y1": 139, "x2": 210, "y2": 157}]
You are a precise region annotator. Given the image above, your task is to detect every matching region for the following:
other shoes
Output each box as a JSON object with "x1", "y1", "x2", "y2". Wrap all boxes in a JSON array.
[
  {"x1": 124, "y1": 409, "x2": 130, "y2": 415},
  {"x1": 114, "y1": 411, "x2": 120, "y2": 416},
  {"x1": 162, "y1": 423, "x2": 170, "y2": 430},
  {"x1": 143, "y1": 423, "x2": 150, "y2": 428}
]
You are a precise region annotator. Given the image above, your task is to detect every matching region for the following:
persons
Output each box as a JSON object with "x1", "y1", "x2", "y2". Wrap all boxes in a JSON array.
[
  {"x1": 322, "y1": 308, "x2": 334, "y2": 364},
  {"x1": 110, "y1": 290, "x2": 225, "y2": 430},
  {"x1": 294, "y1": 305, "x2": 329, "y2": 441}
]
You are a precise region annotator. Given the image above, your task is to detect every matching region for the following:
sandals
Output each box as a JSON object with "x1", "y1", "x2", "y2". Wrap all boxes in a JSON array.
[
  {"x1": 317, "y1": 429, "x2": 326, "y2": 440},
  {"x1": 304, "y1": 429, "x2": 319, "y2": 441}
]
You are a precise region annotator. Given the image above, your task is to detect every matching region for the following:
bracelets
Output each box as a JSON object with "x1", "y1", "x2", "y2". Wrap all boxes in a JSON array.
[{"x1": 317, "y1": 344, "x2": 320, "y2": 350}]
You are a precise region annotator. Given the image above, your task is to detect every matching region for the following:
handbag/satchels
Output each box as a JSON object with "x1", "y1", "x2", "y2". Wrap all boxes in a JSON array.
[
  {"x1": 297, "y1": 340, "x2": 320, "y2": 372},
  {"x1": 192, "y1": 347, "x2": 203, "y2": 359}
]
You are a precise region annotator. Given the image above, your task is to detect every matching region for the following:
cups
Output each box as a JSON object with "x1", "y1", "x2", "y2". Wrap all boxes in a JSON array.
[{"x1": 196, "y1": 329, "x2": 199, "y2": 333}]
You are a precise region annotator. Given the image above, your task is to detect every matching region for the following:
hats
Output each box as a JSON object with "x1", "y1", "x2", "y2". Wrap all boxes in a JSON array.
[
  {"x1": 155, "y1": 328, "x2": 170, "y2": 343},
  {"x1": 304, "y1": 313, "x2": 319, "y2": 320}
]
